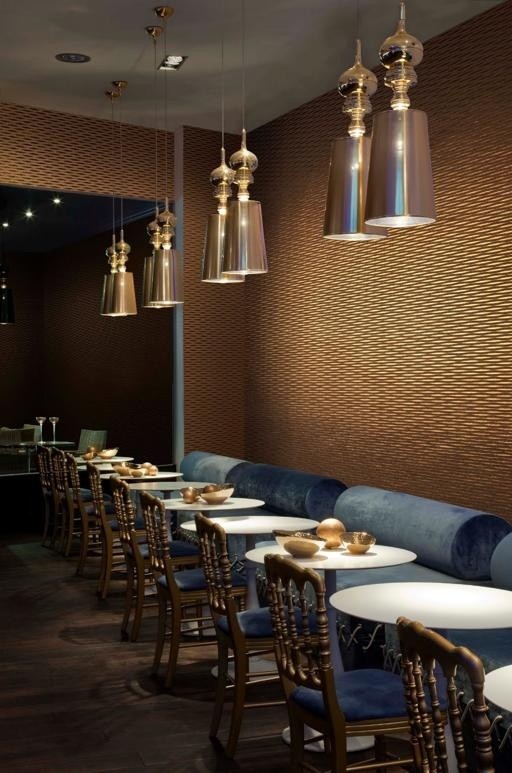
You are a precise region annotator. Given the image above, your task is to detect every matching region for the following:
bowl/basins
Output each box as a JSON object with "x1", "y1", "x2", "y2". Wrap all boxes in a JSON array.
[
  {"x1": 112, "y1": 461, "x2": 141, "y2": 475},
  {"x1": 273, "y1": 529, "x2": 327, "y2": 557},
  {"x1": 339, "y1": 532, "x2": 376, "y2": 553},
  {"x1": 199, "y1": 483, "x2": 234, "y2": 504},
  {"x1": 96, "y1": 450, "x2": 118, "y2": 458}
]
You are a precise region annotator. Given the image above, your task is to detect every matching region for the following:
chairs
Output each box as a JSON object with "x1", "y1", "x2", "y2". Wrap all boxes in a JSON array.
[
  {"x1": 0, "y1": 428, "x2": 35, "y2": 474},
  {"x1": 37, "y1": 446, "x2": 200, "y2": 641},
  {"x1": 78, "y1": 430, "x2": 106, "y2": 454},
  {"x1": 138, "y1": 490, "x2": 246, "y2": 687},
  {"x1": 24, "y1": 424, "x2": 41, "y2": 442},
  {"x1": 191, "y1": 512, "x2": 317, "y2": 771},
  {"x1": 396, "y1": 617, "x2": 511, "y2": 773},
  {"x1": 264, "y1": 553, "x2": 450, "y2": 773}
]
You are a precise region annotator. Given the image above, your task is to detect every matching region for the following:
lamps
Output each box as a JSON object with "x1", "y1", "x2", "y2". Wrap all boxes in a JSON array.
[
  {"x1": 200, "y1": 1, "x2": 247, "y2": 284},
  {"x1": 222, "y1": 1, "x2": 269, "y2": 274},
  {"x1": 99, "y1": 90, "x2": 125, "y2": 317},
  {"x1": 365, "y1": 1, "x2": 436, "y2": 227},
  {"x1": 323, "y1": 1, "x2": 388, "y2": 241},
  {"x1": 147, "y1": 5, "x2": 185, "y2": 304},
  {"x1": 141, "y1": 25, "x2": 175, "y2": 308},
  {"x1": 108, "y1": 80, "x2": 138, "y2": 315}
]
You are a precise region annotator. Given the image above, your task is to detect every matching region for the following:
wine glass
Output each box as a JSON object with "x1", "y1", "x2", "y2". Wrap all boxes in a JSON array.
[
  {"x1": 36, "y1": 417, "x2": 46, "y2": 443},
  {"x1": 49, "y1": 417, "x2": 59, "y2": 442}
]
